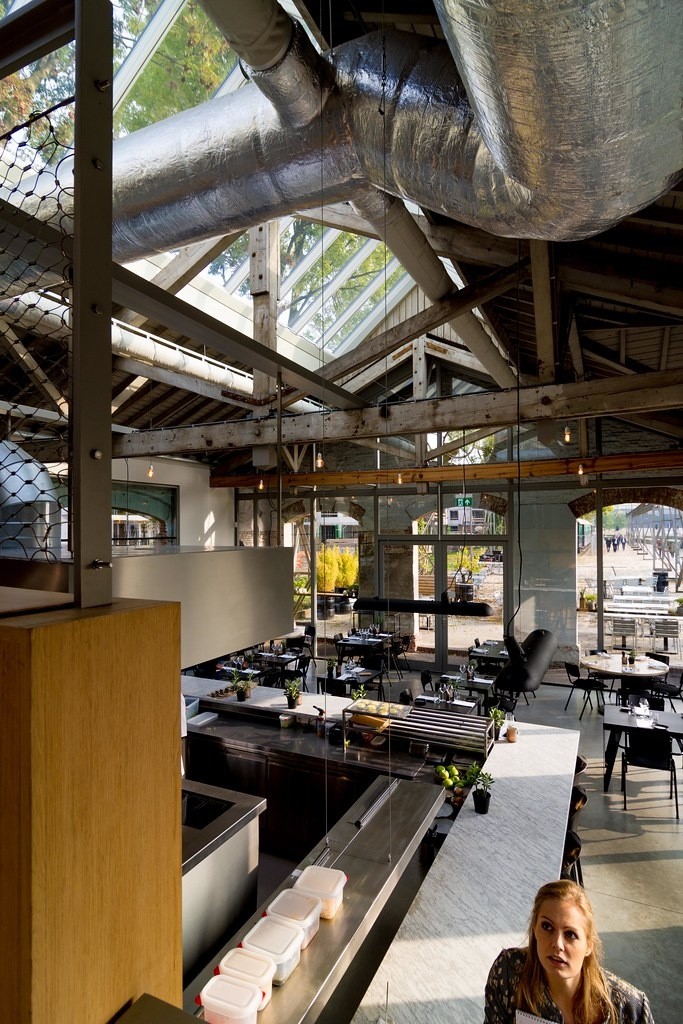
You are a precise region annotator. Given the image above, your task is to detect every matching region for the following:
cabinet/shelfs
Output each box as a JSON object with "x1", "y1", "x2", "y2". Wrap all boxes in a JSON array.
[
  {"x1": 186, "y1": 739, "x2": 378, "y2": 863},
  {"x1": 0, "y1": 586, "x2": 184, "y2": 1024}
]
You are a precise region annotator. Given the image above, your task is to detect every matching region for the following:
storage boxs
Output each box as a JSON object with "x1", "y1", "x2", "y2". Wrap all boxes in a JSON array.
[{"x1": 184, "y1": 696, "x2": 199, "y2": 720}]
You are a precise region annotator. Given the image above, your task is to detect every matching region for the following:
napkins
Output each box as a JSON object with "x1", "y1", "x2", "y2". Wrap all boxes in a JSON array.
[
  {"x1": 636, "y1": 719, "x2": 653, "y2": 729},
  {"x1": 634, "y1": 707, "x2": 650, "y2": 716},
  {"x1": 468, "y1": 677, "x2": 494, "y2": 684},
  {"x1": 450, "y1": 700, "x2": 476, "y2": 708},
  {"x1": 415, "y1": 695, "x2": 439, "y2": 702},
  {"x1": 441, "y1": 675, "x2": 460, "y2": 680}
]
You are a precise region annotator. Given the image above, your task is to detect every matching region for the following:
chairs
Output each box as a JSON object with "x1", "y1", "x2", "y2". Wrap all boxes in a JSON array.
[{"x1": 182, "y1": 618, "x2": 683, "y2": 820}]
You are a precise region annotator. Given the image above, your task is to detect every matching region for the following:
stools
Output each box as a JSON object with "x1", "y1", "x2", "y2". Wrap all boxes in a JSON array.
[{"x1": 560, "y1": 755, "x2": 588, "y2": 888}]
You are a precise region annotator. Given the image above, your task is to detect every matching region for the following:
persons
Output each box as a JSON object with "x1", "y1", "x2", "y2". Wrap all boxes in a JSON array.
[
  {"x1": 668, "y1": 542, "x2": 675, "y2": 557},
  {"x1": 483, "y1": 880, "x2": 654, "y2": 1024},
  {"x1": 657, "y1": 538, "x2": 664, "y2": 555},
  {"x1": 606, "y1": 534, "x2": 626, "y2": 552}
]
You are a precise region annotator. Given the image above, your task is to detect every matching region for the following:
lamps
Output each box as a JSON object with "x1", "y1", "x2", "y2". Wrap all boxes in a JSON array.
[{"x1": 353, "y1": 358, "x2": 560, "y2": 694}]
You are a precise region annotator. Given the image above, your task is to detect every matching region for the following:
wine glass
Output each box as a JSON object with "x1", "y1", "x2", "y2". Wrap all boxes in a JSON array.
[
  {"x1": 233, "y1": 656, "x2": 244, "y2": 672},
  {"x1": 639, "y1": 698, "x2": 649, "y2": 720},
  {"x1": 359, "y1": 624, "x2": 380, "y2": 641},
  {"x1": 459, "y1": 664, "x2": 474, "y2": 681},
  {"x1": 271, "y1": 643, "x2": 282, "y2": 656},
  {"x1": 439, "y1": 682, "x2": 453, "y2": 704}
]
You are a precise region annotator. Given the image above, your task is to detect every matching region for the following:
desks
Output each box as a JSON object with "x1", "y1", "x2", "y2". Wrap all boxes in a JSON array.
[
  {"x1": 317, "y1": 666, "x2": 382, "y2": 701},
  {"x1": 435, "y1": 671, "x2": 497, "y2": 718},
  {"x1": 336, "y1": 630, "x2": 401, "y2": 673},
  {"x1": 580, "y1": 585, "x2": 683, "y2": 792},
  {"x1": 469, "y1": 640, "x2": 522, "y2": 674}
]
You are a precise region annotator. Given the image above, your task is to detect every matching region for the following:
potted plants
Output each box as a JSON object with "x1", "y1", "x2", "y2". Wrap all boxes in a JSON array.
[
  {"x1": 284, "y1": 677, "x2": 301, "y2": 709},
  {"x1": 317, "y1": 542, "x2": 360, "y2": 619},
  {"x1": 488, "y1": 707, "x2": 506, "y2": 741},
  {"x1": 466, "y1": 761, "x2": 495, "y2": 814},
  {"x1": 231, "y1": 668, "x2": 248, "y2": 702},
  {"x1": 244, "y1": 671, "x2": 254, "y2": 697}
]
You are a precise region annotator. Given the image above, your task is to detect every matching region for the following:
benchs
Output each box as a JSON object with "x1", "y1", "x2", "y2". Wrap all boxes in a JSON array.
[{"x1": 418, "y1": 575, "x2": 456, "y2": 603}]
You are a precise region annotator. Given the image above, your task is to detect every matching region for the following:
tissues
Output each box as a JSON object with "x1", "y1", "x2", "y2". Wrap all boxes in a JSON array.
[{"x1": 633, "y1": 702, "x2": 650, "y2": 716}]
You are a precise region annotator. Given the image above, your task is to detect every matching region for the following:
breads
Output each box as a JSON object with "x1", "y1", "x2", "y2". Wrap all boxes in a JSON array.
[{"x1": 356, "y1": 699, "x2": 403, "y2": 715}]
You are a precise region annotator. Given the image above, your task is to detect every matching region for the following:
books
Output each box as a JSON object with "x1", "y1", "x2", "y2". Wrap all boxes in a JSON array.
[{"x1": 516, "y1": 1009, "x2": 559, "y2": 1024}]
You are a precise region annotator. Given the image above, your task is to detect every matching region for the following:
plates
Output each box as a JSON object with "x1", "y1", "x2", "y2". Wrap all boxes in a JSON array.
[
  {"x1": 621, "y1": 706, "x2": 631, "y2": 709},
  {"x1": 654, "y1": 724, "x2": 668, "y2": 729},
  {"x1": 429, "y1": 787, "x2": 454, "y2": 833}
]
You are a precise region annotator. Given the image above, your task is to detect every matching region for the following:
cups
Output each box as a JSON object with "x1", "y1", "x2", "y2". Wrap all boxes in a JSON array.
[
  {"x1": 505, "y1": 713, "x2": 514, "y2": 728},
  {"x1": 345, "y1": 659, "x2": 354, "y2": 671},
  {"x1": 492, "y1": 639, "x2": 496, "y2": 646},
  {"x1": 484, "y1": 647, "x2": 489, "y2": 654}
]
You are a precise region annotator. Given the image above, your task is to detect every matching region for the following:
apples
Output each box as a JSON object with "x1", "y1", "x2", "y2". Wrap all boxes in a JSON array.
[{"x1": 437, "y1": 765, "x2": 464, "y2": 805}]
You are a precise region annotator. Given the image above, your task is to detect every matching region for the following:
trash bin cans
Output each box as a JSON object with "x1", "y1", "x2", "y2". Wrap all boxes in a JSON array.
[
  {"x1": 653, "y1": 572, "x2": 668, "y2": 592},
  {"x1": 455, "y1": 583, "x2": 473, "y2": 601}
]
